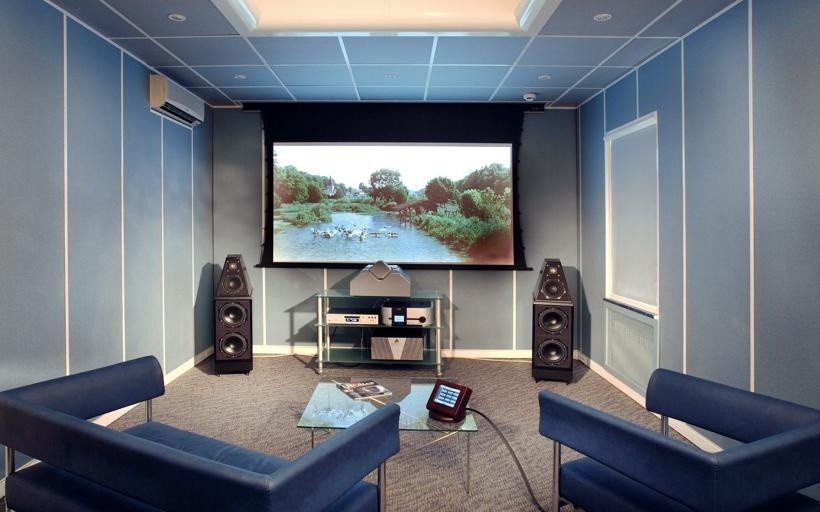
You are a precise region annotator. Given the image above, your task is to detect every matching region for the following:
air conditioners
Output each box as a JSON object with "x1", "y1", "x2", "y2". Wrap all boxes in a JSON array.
[{"x1": 149, "y1": 72, "x2": 205, "y2": 131}]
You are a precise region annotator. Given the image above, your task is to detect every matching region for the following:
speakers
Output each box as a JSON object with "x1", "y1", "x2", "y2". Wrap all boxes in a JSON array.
[
  {"x1": 531, "y1": 258, "x2": 573, "y2": 385},
  {"x1": 213, "y1": 253, "x2": 254, "y2": 376}
]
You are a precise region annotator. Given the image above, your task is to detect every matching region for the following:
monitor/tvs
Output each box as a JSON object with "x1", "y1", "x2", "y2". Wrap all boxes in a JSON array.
[{"x1": 426, "y1": 379, "x2": 472, "y2": 423}]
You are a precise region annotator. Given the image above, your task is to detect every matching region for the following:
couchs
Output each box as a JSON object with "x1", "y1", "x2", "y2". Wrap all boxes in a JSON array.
[{"x1": 0, "y1": 354, "x2": 401, "y2": 511}]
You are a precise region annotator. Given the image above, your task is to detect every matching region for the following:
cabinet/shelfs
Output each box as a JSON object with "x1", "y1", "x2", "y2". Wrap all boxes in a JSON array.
[{"x1": 313, "y1": 290, "x2": 445, "y2": 376}]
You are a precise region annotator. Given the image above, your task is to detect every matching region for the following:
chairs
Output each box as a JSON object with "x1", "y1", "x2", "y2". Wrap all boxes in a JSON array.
[{"x1": 538, "y1": 368, "x2": 820, "y2": 512}]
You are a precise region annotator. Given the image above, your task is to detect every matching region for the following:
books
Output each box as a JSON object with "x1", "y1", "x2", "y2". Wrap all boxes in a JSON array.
[{"x1": 336, "y1": 379, "x2": 394, "y2": 400}]
